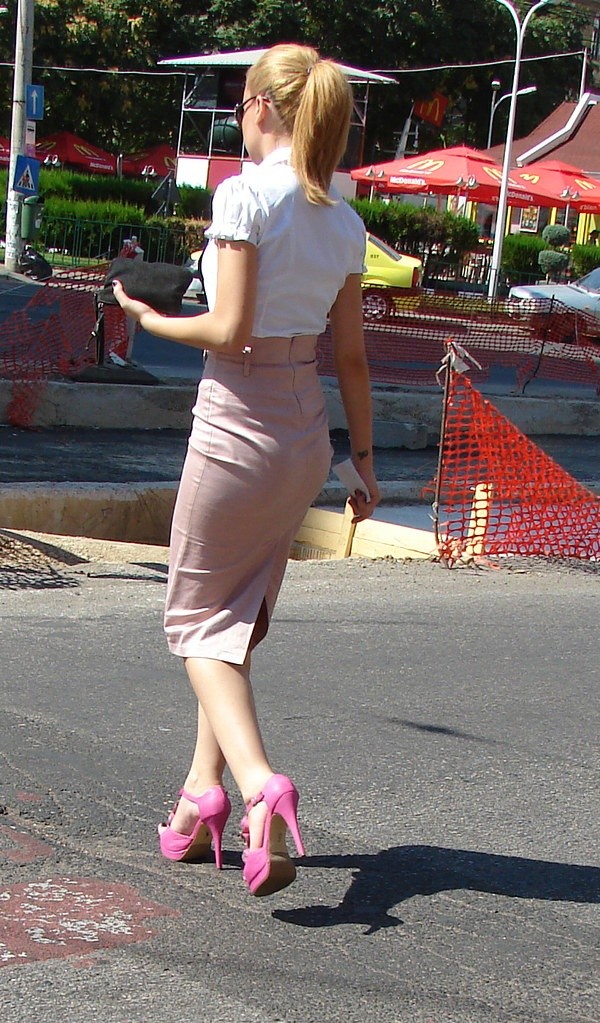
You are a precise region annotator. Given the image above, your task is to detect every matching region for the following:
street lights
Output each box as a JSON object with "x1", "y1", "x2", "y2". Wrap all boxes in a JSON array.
[
  {"x1": 488, "y1": 81, "x2": 537, "y2": 149},
  {"x1": 488, "y1": 0, "x2": 558, "y2": 303}
]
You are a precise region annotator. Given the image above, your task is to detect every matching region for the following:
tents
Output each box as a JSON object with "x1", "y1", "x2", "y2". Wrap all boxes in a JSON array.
[
  {"x1": 350, "y1": 144, "x2": 600, "y2": 237},
  {"x1": 0, "y1": 132, "x2": 177, "y2": 179}
]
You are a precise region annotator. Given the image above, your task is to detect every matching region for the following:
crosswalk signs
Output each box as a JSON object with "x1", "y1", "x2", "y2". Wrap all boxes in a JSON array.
[{"x1": 17, "y1": 164, "x2": 35, "y2": 191}]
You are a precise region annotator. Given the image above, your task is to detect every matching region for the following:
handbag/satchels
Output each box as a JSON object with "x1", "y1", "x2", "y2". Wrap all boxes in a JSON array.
[{"x1": 85, "y1": 257, "x2": 194, "y2": 366}]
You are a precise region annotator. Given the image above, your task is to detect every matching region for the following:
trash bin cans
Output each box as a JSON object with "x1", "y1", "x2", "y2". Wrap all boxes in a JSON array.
[{"x1": 21, "y1": 195, "x2": 45, "y2": 239}]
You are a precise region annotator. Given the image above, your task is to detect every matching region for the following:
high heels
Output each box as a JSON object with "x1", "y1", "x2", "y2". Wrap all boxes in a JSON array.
[
  {"x1": 159, "y1": 785, "x2": 231, "y2": 870},
  {"x1": 241, "y1": 773, "x2": 305, "y2": 897}
]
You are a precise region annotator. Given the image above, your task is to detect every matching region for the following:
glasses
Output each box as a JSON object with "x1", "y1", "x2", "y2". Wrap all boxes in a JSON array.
[{"x1": 234, "y1": 95, "x2": 270, "y2": 131}]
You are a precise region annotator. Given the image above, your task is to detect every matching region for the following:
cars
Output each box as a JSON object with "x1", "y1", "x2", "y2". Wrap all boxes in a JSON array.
[
  {"x1": 185, "y1": 232, "x2": 425, "y2": 324},
  {"x1": 504, "y1": 267, "x2": 600, "y2": 343}
]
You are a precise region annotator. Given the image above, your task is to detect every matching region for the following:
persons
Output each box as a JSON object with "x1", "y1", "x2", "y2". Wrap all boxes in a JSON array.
[
  {"x1": 588, "y1": 230, "x2": 600, "y2": 246},
  {"x1": 113, "y1": 41, "x2": 380, "y2": 897},
  {"x1": 482, "y1": 212, "x2": 493, "y2": 236}
]
